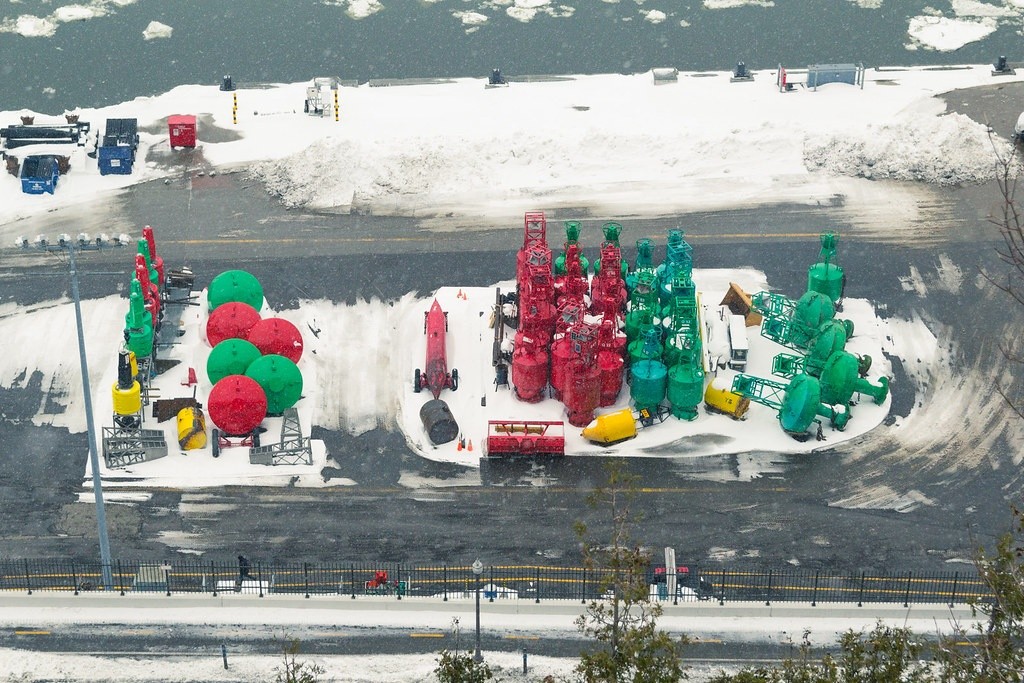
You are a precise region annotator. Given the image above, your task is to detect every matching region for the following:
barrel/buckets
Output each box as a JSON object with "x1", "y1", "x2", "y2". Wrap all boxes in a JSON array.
[
  {"x1": 705, "y1": 378, "x2": 749, "y2": 418},
  {"x1": 177, "y1": 407, "x2": 207, "y2": 451},
  {"x1": 420, "y1": 399, "x2": 459, "y2": 444}
]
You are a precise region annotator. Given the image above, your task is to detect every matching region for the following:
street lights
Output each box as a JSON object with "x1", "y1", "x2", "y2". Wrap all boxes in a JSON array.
[{"x1": 471, "y1": 558, "x2": 484, "y2": 665}]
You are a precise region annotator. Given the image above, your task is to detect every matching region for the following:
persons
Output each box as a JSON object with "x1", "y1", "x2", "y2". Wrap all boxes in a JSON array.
[{"x1": 237, "y1": 555, "x2": 256, "y2": 583}]
